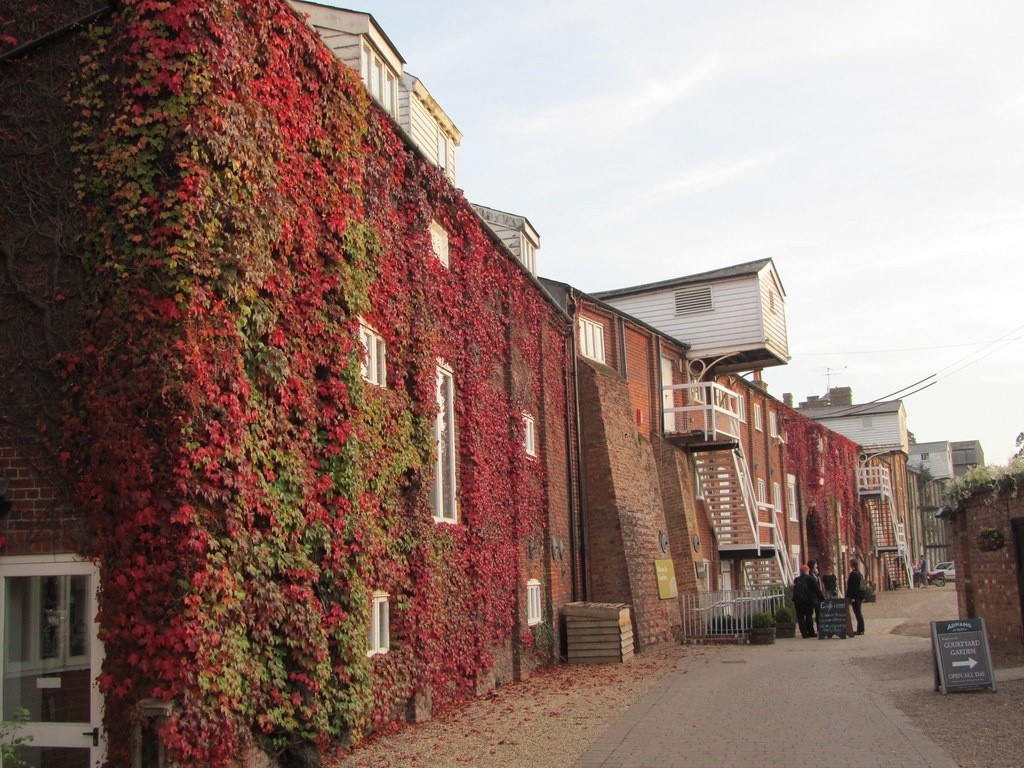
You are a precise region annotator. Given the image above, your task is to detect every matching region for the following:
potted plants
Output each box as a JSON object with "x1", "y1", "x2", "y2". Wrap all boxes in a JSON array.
[
  {"x1": 864, "y1": 585, "x2": 876, "y2": 601},
  {"x1": 746, "y1": 609, "x2": 778, "y2": 645},
  {"x1": 773, "y1": 607, "x2": 796, "y2": 638}
]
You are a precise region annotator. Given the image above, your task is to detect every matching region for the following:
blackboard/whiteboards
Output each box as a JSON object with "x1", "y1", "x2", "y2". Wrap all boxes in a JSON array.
[{"x1": 818, "y1": 598, "x2": 853, "y2": 634}]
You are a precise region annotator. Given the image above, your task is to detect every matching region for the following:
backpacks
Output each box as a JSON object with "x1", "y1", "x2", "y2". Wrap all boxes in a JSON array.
[{"x1": 854, "y1": 571, "x2": 866, "y2": 593}]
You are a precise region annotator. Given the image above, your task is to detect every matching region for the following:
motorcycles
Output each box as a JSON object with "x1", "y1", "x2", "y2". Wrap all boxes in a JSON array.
[{"x1": 912, "y1": 570, "x2": 946, "y2": 587}]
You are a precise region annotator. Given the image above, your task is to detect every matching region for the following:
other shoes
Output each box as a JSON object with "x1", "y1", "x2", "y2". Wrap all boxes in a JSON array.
[
  {"x1": 855, "y1": 631, "x2": 864, "y2": 635},
  {"x1": 808, "y1": 634, "x2": 817, "y2": 637},
  {"x1": 802, "y1": 634, "x2": 807, "y2": 638}
]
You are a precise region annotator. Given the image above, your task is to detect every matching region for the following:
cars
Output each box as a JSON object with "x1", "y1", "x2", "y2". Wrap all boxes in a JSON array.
[{"x1": 930, "y1": 561, "x2": 955, "y2": 582}]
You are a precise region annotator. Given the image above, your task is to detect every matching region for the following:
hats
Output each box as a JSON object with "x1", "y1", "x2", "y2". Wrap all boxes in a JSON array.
[{"x1": 800, "y1": 565, "x2": 809, "y2": 572}]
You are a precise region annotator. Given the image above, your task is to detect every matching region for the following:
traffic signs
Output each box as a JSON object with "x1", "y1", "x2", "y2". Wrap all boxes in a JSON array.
[{"x1": 929, "y1": 616, "x2": 999, "y2": 695}]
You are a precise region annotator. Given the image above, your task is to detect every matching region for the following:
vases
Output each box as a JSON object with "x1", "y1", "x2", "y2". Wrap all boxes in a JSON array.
[{"x1": 974, "y1": 535, "x2": 1004, "y2": 552}]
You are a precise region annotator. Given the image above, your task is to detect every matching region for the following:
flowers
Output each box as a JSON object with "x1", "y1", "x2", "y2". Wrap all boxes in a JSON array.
[{"x1": 973, "y1": 527, "x2": 1004, "y2": 539}]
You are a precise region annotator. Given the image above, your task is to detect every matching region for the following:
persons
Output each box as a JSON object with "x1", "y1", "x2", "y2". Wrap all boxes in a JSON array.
[
  {"x1": 793, "y1": 560, "x2": 837, "y2": 637},
  {"x1": 920, "y1": 555, "x2": 929, "y2": 588},
  {"x1": 848, "y1": 559, "x2": 865, "y2": 635}
]
